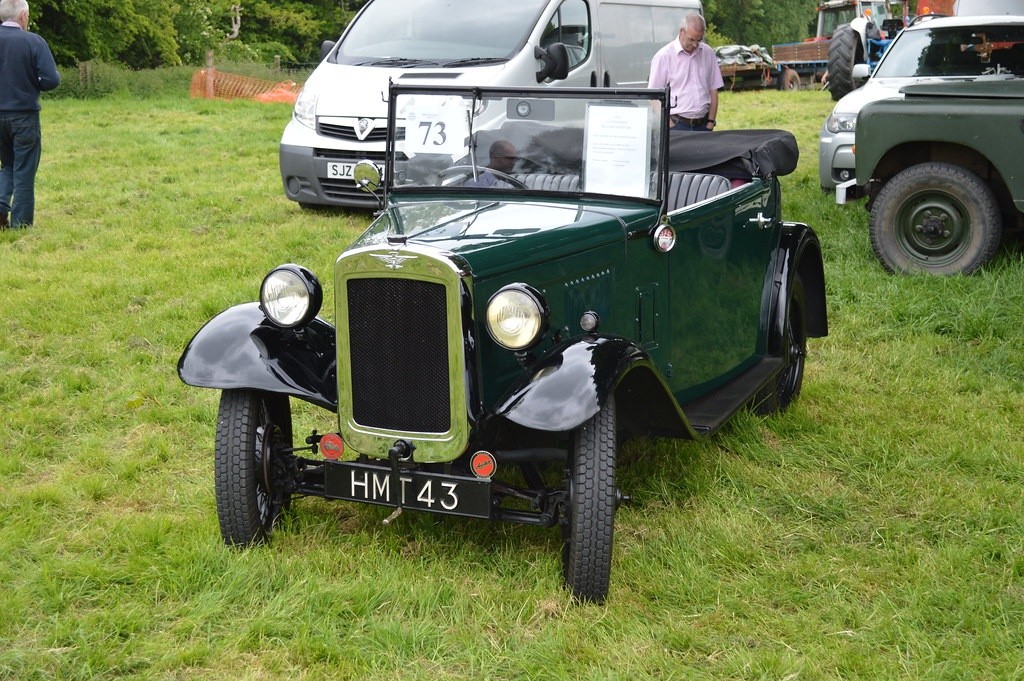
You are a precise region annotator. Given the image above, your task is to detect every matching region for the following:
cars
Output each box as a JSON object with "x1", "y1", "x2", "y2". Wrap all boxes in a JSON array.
[{"x1": 175, "y1": 77, "x2": 830, "y2": 604}]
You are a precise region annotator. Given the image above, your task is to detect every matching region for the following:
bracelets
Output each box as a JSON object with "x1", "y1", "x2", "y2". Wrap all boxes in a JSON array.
[{"x1": 707, "y1": 119, "x2": 716, "y2": 126}]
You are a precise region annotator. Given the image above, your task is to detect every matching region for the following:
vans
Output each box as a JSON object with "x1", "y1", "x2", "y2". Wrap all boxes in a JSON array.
[{"x1": 278, "y1": 0, "x2": 704, "y2": 210}]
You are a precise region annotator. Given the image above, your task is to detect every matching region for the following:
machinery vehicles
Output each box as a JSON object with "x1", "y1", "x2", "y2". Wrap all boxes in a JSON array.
[{"x1": 827, "y1": 1, "x2": 921, "y2": 101}]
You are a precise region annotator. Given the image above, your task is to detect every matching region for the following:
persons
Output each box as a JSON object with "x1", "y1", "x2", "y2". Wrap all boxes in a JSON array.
[
  {"x1": 648, "y1": 13, "x2": 724, "y2": 131},
  {"x1": 0, "y1": 0, "x2": 61, "y2": 227},
  {"x1": 464, "y1": 140, "x2": 518, "y2": 187}
]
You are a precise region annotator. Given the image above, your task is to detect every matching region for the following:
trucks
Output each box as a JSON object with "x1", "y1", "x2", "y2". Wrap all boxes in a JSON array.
[{"x1": 772, "y1": 0, "x2": 907, "y2": 89}]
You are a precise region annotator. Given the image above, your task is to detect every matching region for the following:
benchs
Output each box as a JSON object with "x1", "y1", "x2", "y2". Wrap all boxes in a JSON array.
[{"x1": 508, "y1": 171, "x2": 732, "y2": 214}]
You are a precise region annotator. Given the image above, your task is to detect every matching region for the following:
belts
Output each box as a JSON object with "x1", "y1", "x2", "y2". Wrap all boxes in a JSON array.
[{"x1": 670, "y1": 113, "x2": 709, "y2": 127}]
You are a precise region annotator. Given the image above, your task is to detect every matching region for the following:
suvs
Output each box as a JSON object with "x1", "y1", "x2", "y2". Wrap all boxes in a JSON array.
[
  {"x1": 832, "y1": 80, "x2": 1023, "y2": 278},
  {"x1": 819, "y1": 13, "x2": 1024, "y2": 192}
]
